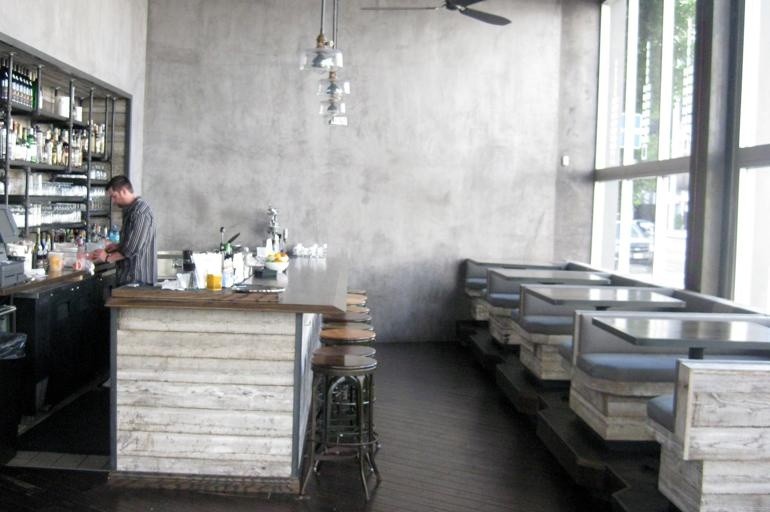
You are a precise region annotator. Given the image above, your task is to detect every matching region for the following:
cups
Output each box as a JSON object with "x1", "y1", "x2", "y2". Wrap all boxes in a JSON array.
[
  {"x1": 307, "y1": 244, "x2": 317, "y2": 259},
  {"x1": 293, "y1": 244, "x2": 305, "y2": 259},
  {"x1": 316, "y1": 243, "x2": 328, "y2": 259}
]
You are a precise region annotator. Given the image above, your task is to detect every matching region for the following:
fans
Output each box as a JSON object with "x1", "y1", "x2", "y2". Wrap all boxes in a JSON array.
[{"x1": 363, "y1": 1, "x2": 515, "y2": 25}]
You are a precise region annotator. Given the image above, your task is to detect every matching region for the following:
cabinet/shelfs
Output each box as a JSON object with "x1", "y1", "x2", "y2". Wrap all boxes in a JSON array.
[
  {"x1": 80, "y1": 262, "x2": 127, "y2": 415},
  {"x1": 10, "y1": 276, "x2": 79, "y2": 439},
  {"x1": 0, "y1": 32, "x2": 132, "y2": 270}
]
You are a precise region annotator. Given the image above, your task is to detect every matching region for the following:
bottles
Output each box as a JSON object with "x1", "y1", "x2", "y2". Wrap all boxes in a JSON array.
[
  {"x1": 170, "y1": 226, "x2": 293, "y2": 293},
  {"x1": 0, "y1": 53, "x2": 122, "y2": 280}
]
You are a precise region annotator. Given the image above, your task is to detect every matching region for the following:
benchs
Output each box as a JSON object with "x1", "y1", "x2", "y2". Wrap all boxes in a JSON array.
[{"x1": 453, "y1": 258, "x2": 769, "y2": 512}]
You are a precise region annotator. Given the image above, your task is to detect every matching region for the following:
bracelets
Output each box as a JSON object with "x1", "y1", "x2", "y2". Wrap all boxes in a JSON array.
[{"x1": 105, "y1": 254, "x2": 110, "y2": 264}]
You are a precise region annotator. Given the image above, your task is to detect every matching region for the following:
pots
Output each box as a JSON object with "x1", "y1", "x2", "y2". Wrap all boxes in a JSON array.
[{"x1": 0, "y1": 305, "x2": 17, "y2": 338}]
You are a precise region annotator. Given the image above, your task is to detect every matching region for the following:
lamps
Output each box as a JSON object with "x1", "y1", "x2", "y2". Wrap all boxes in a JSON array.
[{"x1": 295, "y1": 1, "x2": 352, "y2": 129}]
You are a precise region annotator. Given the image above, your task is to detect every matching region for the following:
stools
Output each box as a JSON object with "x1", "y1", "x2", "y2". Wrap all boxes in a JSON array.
[{"x1": 297, "y1": 288, "x2": 384, "y2": 502}]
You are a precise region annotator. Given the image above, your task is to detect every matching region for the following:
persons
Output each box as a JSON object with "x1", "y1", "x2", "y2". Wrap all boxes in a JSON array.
[{"x1": 87, "y1": 173, "x2": 160, "y2": 391}]
